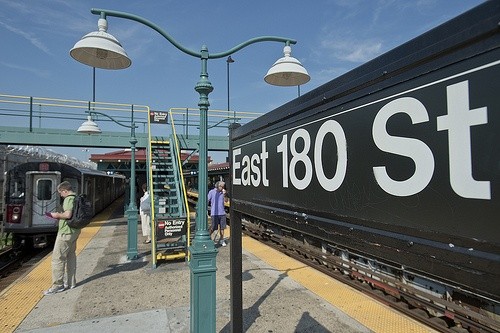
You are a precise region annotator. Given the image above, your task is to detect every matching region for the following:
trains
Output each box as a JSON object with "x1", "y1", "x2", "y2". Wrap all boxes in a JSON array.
[
  {"x1": 187, "y1": 0, "x2": 500, "y2": 333},
  {"x1": 2, "y1": 160, "x2": 127, "y2": 256}
]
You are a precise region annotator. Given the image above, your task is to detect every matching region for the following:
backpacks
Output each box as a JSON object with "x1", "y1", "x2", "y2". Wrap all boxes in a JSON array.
[{"x1": 68, "y1": 194, "x2": 92, "y2": 228}]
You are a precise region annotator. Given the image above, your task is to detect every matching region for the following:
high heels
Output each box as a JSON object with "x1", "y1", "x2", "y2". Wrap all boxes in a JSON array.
[{"x1": 143, "y1": 240, "x2": 150, "y2": 244}]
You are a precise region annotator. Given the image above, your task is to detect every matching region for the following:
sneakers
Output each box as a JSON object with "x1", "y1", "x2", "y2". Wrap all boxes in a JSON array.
[
  {"x1": 45, "y1": 287, "x2": 63, "y2": 294},
  {"x1": 66, "y1": 282, "x2": 76, "y2": 288},
  {"x1": 219, "y1": 240, "x2": 226, "y2": 246}
]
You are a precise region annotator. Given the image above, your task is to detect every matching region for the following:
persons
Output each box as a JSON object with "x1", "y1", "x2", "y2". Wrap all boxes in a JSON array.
[
  {"x1": 140, "y1": 185, "x2": 154, "y2": 244},
  {"x1": 208, "y1": 181, "x2": 228, "y2": 246},
  {"x1": 43, "y1": 182, "x2": 81, "y2": 295}
]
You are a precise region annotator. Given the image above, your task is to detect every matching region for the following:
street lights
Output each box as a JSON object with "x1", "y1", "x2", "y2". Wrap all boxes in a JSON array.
[
  {"x1": 225, "y1": 56, "x2": 235, "y2": 124},
  {"x1": 69, "y1": 7, "x2": 311, "y2": 333},
  {"x1": 76, "y1": 110, "x2": 139, "y2": 260}
]
